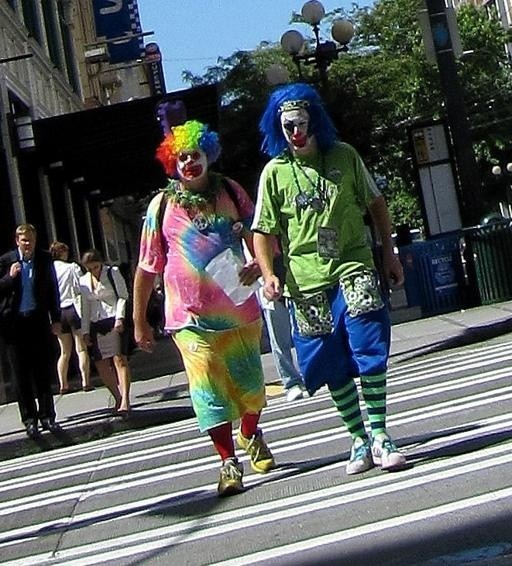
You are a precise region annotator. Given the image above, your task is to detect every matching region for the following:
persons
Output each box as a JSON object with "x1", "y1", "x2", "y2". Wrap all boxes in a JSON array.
[
  {"x1": 131, "y1": 118, "x2": 279, "y2": 495},
  {"x1": 0, "y1": 225, "x2": 62, "y2": 439},
  {"x1": 251, "y1": 85, "x2": 405, "y2": 475},
  {"x1": 240, "y1": 236, "x2": 330, "y2": 400},
  {"x1": 45, "y1": 241, "x2": 95, "y2": 395},
  {"x1": 80, "y1": 253, "x2": 133, "y2": 415}
]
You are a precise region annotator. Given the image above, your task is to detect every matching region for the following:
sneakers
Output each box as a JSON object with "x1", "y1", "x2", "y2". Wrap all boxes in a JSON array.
[
  {"x1": 218, "y1": 459, "x2": 244, "y2": 497},
  {"x1": 346, "y1": 435, "x2": 374, "y2": 475},
  {"x1": 236, "y1": 424, "x2": 276, "y2": 473},
  {"x1": 285, "y1": 385, "x2": 311, "y2": 402},
  {"x1": 371, "y1": 430, "x2": 406, "y2": 470}
]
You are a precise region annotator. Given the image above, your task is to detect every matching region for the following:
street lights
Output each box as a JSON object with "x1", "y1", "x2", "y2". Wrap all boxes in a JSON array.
[
  {"x1": 267, "y1": 1, "x2": 355, "y2": 129},
  {"x1": 491, "y1": 163, "x2": 511, "y2": 203}
]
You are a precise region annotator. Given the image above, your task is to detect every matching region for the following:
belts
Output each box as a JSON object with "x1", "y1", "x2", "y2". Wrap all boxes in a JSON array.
[{"x1": 19, "y1": 310, "x2": 36, "y2": 319}]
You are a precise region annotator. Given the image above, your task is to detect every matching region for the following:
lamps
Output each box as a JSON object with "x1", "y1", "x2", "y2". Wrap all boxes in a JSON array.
[{"x1": 8, "y1": 107, "x2": 36, "y2": 157}]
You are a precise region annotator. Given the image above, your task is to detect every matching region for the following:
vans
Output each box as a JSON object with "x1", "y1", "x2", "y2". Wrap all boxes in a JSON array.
[{"x1": 392, "y1": 228, "x2": 421, "y2": 254}]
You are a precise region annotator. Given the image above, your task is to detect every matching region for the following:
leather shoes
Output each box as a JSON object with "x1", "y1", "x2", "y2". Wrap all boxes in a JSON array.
[
  {"x1": 26, "y1": 423, "x2": 39, "y2": 436},
  {"x1": 42, "y1": 421, "x2": 61, "y2": 433},
  {"x1": 60, "y1": 384, "x2": 94, "y2": 394},
  {"x1": 110, "y1": 401, "x2": 131, "y2": 416}
]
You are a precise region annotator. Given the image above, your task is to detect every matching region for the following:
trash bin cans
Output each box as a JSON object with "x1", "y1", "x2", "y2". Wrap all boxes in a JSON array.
[{"x1": 397, "y1": 217, "x2": 512, "y2": 317}]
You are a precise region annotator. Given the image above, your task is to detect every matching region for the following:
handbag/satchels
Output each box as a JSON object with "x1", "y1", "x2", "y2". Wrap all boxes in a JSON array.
[{"x1": 118, "y1": 313, "x2": 142, "y2": 357}]
